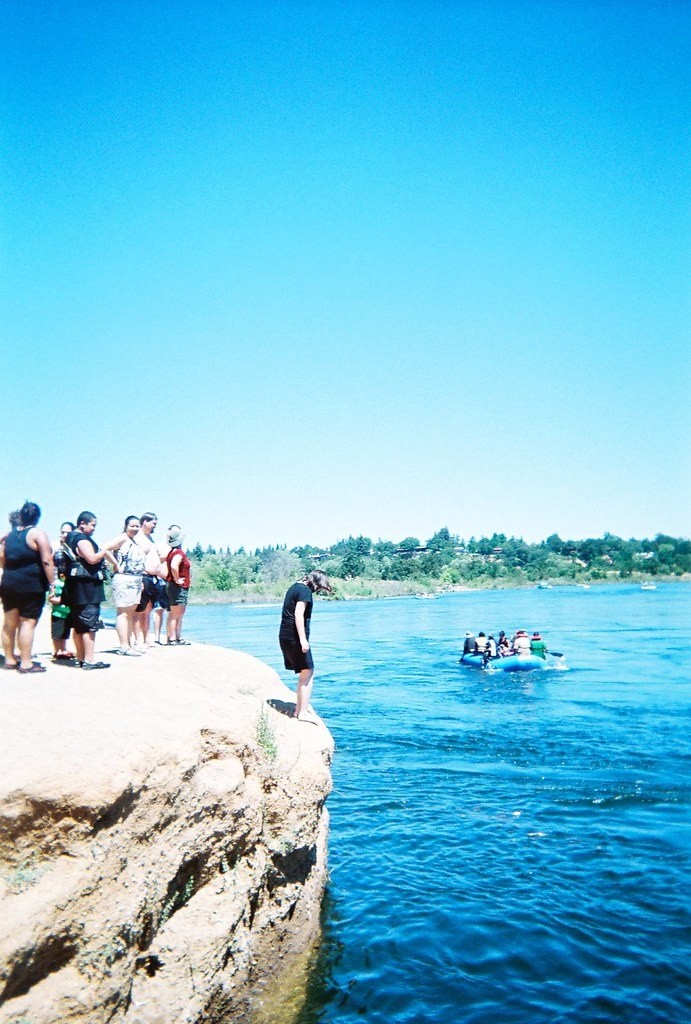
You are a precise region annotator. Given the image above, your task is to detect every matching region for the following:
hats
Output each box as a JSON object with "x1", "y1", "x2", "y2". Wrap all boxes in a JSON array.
[
  {"x1": 464, "y1": 631, "x2": 474, "y2": 639},
  {"x1": 516, "y1": 629, "x2": 528, "y2": 636},
  {"x1": 531, "y1": 632, "x2": 540, "y2": 639},
  {"x1": 165, "y1": 527, "x2": 185, "y2": 547}
]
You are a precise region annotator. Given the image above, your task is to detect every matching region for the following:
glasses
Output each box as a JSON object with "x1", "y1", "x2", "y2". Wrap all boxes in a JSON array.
[{"x1": 61, "y1": 531, "x2": 69, "y2": 535}]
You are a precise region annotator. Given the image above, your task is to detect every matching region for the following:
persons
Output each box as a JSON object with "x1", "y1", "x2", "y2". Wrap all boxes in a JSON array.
[
  {"x1": 463, "y1": 629, "x2": 548, "y2": 660},
  {"x1": 279, "y1": 569, "x2": 332, "y2": 725},
  {"x1": 0, "y1": 497, "x2": 190, "y2": 673}
]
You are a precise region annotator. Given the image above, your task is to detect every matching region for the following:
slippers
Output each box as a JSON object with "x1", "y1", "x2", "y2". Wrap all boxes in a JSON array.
[
  {"x1": 18, "y1": 665, "x2": 45, "y2": 673},
  {"x1": 5, "y1": 660, "x2": 21, "y2": 668}
]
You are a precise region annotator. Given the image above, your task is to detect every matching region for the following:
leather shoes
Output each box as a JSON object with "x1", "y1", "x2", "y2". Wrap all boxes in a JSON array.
[
  {"x1": 83, "y1": 661, "x2": 111, "y2": 670},
  {"x1": 72, "y1": 660, "x2": 84, "y2": 668}
]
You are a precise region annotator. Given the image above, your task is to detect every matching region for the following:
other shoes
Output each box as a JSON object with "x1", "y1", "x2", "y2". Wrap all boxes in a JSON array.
[
  {"x1": 117, "y1": 637, "x2": 192, "y2": 655},
  {"x1": 54, "y1": 652, "x2": 75, "y2": 660}
]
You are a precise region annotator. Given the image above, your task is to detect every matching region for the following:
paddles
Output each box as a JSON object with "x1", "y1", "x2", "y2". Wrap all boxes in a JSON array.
[{"x1": 544, "y1": 651, "x2": 563, "y2": 657}]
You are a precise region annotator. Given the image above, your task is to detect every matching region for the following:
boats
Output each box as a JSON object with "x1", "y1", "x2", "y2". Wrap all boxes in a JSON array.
[
  {"x1": 641, "y1": 583, "x2": 657, "y2": 589},
  {"x1": 538, "y1": 584, "x2": 548, "y2": 589},
  {"x1": 462, "y1": 651, "x2": 564, "y2": 675}
]
